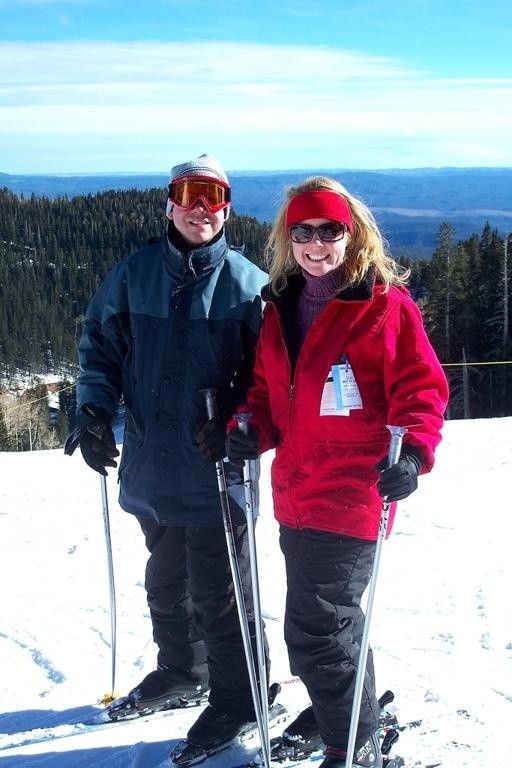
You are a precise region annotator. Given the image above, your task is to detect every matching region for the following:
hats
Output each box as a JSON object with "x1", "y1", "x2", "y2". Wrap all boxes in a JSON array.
[{"x1": 166, "y1": 154, "x2": 230, "y2": 222}]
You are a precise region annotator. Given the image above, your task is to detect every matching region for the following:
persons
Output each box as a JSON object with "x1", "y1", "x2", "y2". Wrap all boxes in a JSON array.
[
  {"x1": 225, "y1": 177, "x2": 450, "y2": 767},
  {"x1": 74, "y1": 153, "x2": 282, "y2": 746}
]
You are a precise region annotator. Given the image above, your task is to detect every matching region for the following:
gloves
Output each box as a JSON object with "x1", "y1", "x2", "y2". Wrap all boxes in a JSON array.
[
  {"x1": 79, "y1": 421, "x2": 119, "y2": 476},
  {"x1": 374, "y1": 443, "x2": 424, "y2": 503},
  {"x1": 225, "y1": 423, "x2": 261, "y2": 467},
  {"x1": 194, "y1": 409, "x2": 232, "y2": 462}
]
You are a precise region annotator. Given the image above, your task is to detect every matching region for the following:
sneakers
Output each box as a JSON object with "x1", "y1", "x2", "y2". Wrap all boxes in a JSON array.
[
  {"x1": 282, "y1": 705, "x2": 323, "y2": 751},
  {"x1": 318, "y1": 733, "x2": 383, "y2": 768},
  {"x1": 128, "y1": 669, "x2": 210, "y2": 710},
  {"x1": 187, "y1": 705, "x2": 248, "y2": 751}
]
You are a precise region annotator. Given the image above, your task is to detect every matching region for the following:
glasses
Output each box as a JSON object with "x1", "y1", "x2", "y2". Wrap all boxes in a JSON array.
[
  {"x1": 168, "y1": 175, "x2": 231, "y2": 214},
  {"x1": 290, "y1": 221, "x2": 346, "y2": 243}
]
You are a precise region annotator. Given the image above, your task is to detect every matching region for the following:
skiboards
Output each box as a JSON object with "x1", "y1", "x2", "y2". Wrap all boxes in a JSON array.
[
  {"x1": 0, "y1": 689, "x2": 312, "y2": 768},
  {"x1": 230, "y1": 693, "x2": 444, "y2": 767}
]
